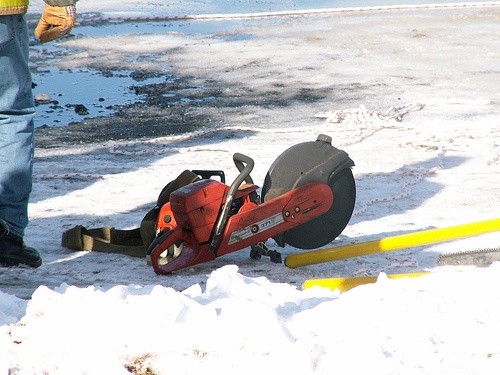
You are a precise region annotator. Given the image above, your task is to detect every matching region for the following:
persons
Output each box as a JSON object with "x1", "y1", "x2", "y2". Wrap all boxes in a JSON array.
[{"x1": 0, "y1": 0, "x2": 79, "y2": 268}]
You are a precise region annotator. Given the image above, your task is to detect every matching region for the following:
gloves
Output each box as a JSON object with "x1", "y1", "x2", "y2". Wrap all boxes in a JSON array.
[{"x1": 34, "y1": 1, "x2": 78, "y2": 43}]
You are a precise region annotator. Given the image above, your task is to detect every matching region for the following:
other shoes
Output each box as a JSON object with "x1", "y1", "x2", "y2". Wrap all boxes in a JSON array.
[{"x1": 0, "y1": 227, "x2": 41, "y2": 268}]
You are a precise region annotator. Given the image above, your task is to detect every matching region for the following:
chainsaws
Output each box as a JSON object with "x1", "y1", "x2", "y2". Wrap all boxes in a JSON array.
[{"x1": 145, "y1": 133, "x2": 358, "y2": 276}]
